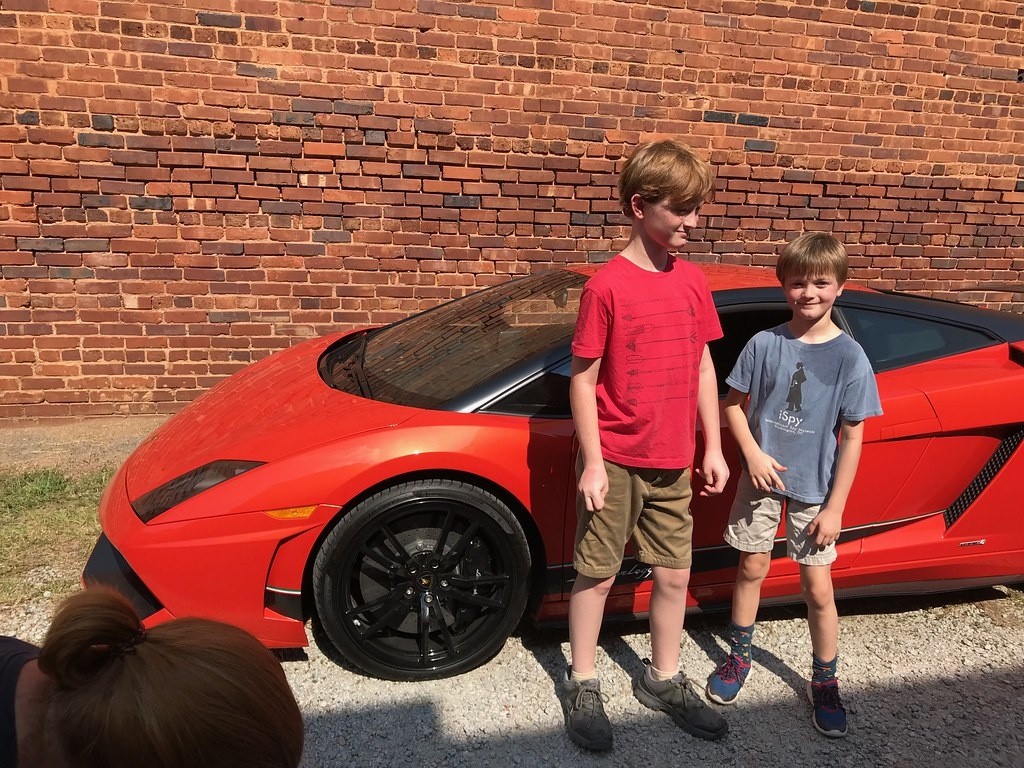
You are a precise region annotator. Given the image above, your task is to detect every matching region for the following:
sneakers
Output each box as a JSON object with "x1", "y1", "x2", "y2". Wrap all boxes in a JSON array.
[
  {"x1": 805, "y1": 679, "x2": 848, "y2": 738},
  {"x1": 632, "y1": 657, "x2": 728, "y2": 740},
  {"x1": 705, "y1": 654, "x2": 751, "y2": 704},
  {"x1": 559, "y1": 665, "x2": 614, "y2": 752}
]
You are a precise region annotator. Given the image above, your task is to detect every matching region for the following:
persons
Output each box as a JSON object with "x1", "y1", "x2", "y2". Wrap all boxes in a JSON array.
[
  {"x1": 702, "y1": 228, "x2": 886, "y2": 741},
  {"x1": 0, "y1": 578, "x2": 305, "y2": 768},
  {"x1": 560, "y1": 139, "x2": 731, "y2": 755}
]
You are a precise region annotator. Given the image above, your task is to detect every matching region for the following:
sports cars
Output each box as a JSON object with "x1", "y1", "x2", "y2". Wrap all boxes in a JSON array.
[{"x1": 98, "y1": 255, "x2": 1023, "y2": 681}]
